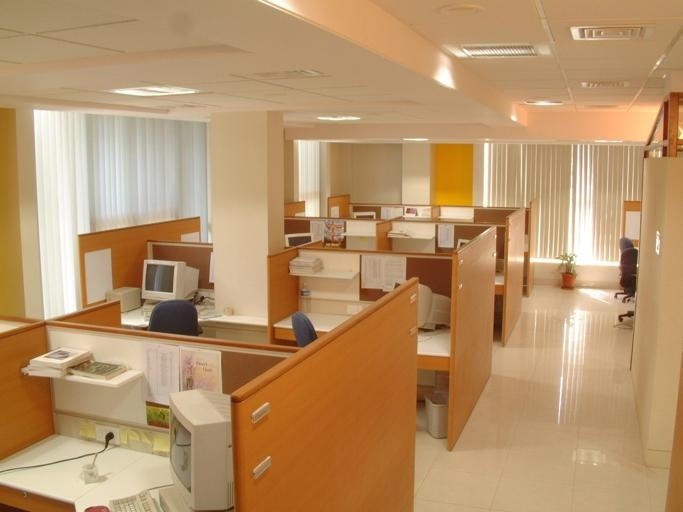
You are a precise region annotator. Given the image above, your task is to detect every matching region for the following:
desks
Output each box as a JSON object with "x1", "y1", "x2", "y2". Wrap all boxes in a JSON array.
[
  {"x1": 1, "y1": 276, "x2": 419, "y2": 512},
  {"x1": 269, "y1": 194, "x2": 535, "y2": 451},
  {"x1": 65, "y1": 216, "x2": 212, "y2": 335}
]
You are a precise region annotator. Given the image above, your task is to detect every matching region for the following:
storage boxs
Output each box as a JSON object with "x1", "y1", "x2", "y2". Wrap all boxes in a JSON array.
[{"x1": 107, "y1": 286, "x2": 141, "y2": 312}]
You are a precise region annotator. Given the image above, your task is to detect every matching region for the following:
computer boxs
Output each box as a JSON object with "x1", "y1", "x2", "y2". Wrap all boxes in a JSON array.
[
  {"x1": 142, "y1": 301, "x2": 160, "y2": 311},
  {"x1": 159, "y1": 485, "x2": 191, "y2": 512}
]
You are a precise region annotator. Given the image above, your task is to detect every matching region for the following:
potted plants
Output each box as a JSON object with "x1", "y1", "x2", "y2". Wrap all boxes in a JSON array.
[{"x1": 554, "y1": 254, "x2": 577, "y2": 289}]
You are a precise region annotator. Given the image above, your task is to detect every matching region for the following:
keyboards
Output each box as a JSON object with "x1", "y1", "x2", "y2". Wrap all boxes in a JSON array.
[
  {"x1": 108, "y1": 489, "x2": 159, "y2": 512},
  {"x1": 144, "y1": 311, "x2": 151, "y2": 321}
]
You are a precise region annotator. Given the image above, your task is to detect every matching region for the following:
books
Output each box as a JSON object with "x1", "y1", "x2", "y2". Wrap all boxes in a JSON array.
[
  {"x1": 65, "y1": 360, "x2": 124, "y2": 379},
  {"x1": 386, "y1": 230, "x2": 407, "y2": 237},
  {"x1": 287, "y1": 256, "x2": 322, "y2": 274},
  {"x1": 20, "y1": 345, "x2": 90, "y2": 378}
]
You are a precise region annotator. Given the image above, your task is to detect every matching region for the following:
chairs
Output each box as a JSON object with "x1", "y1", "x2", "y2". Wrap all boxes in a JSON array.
[{"x1": 614, "y1": 236, "x2": 638, "y2": 322}]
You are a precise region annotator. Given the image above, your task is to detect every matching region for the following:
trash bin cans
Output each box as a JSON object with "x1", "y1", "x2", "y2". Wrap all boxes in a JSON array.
[{"x1": 424, "y1": 391, "x2": 449, "y2": 439}]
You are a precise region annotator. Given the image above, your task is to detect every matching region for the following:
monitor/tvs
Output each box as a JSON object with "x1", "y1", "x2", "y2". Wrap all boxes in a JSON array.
[
  {"x1": 352, "y1": 211, "x2": 376, "y2": 219},
  {"x1": 285, "y1": 232, "x2": 313, "y2": 247},
  {"x1": 457, "y1": 239, "x2": 470, "y2": 248},
  {"x1": 141, "y1": 260, "x2": 200, "y2": 301},
  {"x1": 391, "y1": 280, "x2": 451, "y2": 331},
  {"x1": 167, "y1": 388, "x2": 233, "y2": 512}
]
